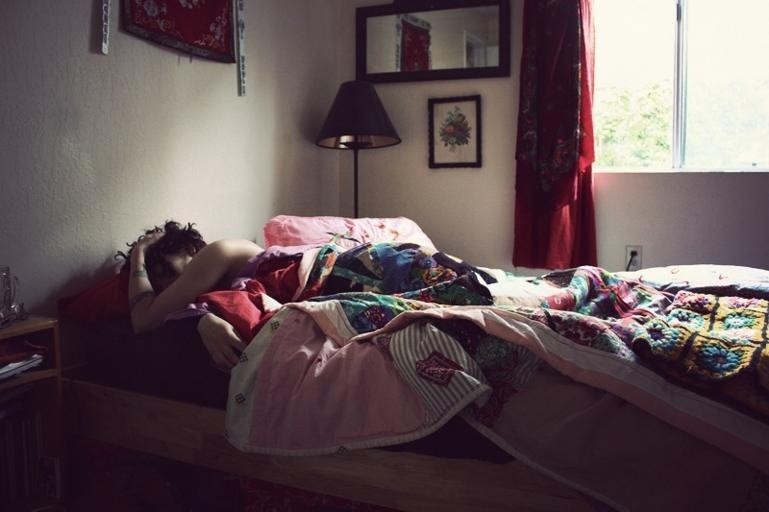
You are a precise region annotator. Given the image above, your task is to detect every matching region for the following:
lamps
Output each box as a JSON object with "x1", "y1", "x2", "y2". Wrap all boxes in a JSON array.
[{"x1": 312, "y1": 80, "x2": 403, "y2": 217}]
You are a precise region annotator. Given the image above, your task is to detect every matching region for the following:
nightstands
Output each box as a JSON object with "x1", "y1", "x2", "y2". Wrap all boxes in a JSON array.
[{"x1": 0, "y1": 316, "x2": 66, "y2": 510}]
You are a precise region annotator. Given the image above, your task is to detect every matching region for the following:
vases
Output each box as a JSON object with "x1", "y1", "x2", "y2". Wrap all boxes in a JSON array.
[{"x1": 454, "y1": 143, "x2": 463, "y2": 162}]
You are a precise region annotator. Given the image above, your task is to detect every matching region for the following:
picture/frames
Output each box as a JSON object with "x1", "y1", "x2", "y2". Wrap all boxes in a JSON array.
[
  {"x1": 118, "y1": 0, "x2": 240, "y2": 65},
  {"x1": 427, "y1": 94, "x2": 482, "y2": 169},
  {"x1": 392, "y1": 12, "x2": 433, "y2": 73}
]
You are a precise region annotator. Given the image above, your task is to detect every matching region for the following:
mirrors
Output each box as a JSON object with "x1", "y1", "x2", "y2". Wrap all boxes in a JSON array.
[{"x1": 355, "y1": 1, "x2": 510, "y2": 84}]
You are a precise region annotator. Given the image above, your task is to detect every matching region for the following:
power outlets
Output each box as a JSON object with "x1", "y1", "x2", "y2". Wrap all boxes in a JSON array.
[{"x1": 625, "y1": 245, "x2": 642, "y2": 271}]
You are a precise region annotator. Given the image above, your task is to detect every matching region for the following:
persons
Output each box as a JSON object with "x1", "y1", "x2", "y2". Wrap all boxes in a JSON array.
[{"x1": 115, "y1": 221, "x2": 265, "y2": 375}]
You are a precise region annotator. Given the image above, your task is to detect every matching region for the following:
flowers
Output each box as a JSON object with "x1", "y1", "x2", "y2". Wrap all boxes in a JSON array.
[{"x1": 440, "y1": 107, "x2": 472, "y2": 147}]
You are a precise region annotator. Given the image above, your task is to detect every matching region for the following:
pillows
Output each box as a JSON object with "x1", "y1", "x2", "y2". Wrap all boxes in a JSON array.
[
  {"x1": 263, "y1": 213, "x2": 438, "y2": 258},
  {"x1": 61, "y1": 270, "x2": 175, "y2": 319}
]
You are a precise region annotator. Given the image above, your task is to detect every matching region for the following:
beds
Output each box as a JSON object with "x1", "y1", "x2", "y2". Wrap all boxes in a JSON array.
[{"x1": 60, "y1": 257, "x2": 769, "y2": 511}]
